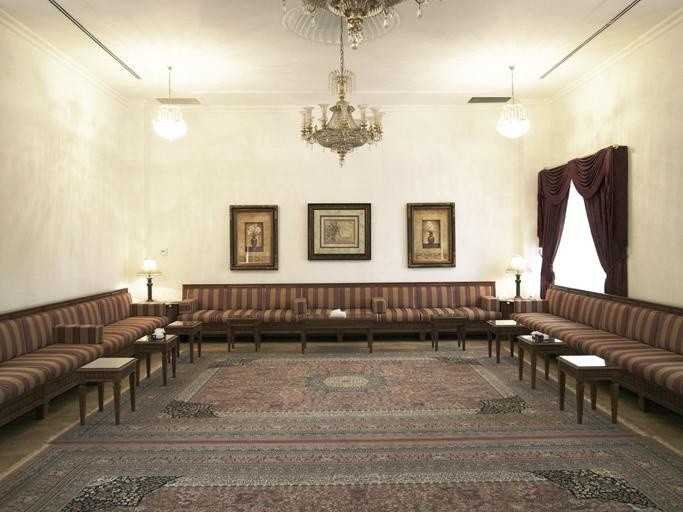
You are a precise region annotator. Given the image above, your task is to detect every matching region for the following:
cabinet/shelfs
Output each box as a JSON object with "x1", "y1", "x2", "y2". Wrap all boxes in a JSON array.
[
  {"x1": 166, "y1": 301, "x2": 178, "y2": 324},
  {"x1": 500, "y1": 300, "x2": 513, "y2": 320}
]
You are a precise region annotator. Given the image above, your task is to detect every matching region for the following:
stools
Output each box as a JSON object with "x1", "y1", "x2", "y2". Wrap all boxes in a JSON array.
[
  {"x1": 556, "y1": 353, "x2": 624, "y2": 426},
  {"x1": 515, "y1": 331, "x2": 568, "y2": 389},
  {"x1": 165, "y1": 320, "x2": 202, "y2": 362},
  {"x1": 225, "y1": 315, "x2": 263, "y2": 353},
  {"x1": 428, "y1": 314, "x2": 468, "y2": 351},
  {"x1": 77, "y1": 357, "x2": 137, "y2": 427},
  {"x1": 133, "y1": 334, "x2": 179, "y2": 386},
  {"x1": 487, "y1": 318, "x2": 526, "y2": 364}
]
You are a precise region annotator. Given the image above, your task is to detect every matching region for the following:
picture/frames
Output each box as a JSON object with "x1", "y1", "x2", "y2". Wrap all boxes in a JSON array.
[
  {"x1": 228, "y1": 205, "x2": 277, "y2": 270},
  {"x1": 309, "y1": 203, "x2": 370, "y2": 261},
  {"x1": 407, "y1": 203, "x2": 455, "y2": 268}
]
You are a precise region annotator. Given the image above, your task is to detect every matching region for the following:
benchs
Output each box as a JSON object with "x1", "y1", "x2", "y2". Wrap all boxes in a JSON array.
[{"x1": 294, "y1": 316, "x2": 378, "y2": 354}]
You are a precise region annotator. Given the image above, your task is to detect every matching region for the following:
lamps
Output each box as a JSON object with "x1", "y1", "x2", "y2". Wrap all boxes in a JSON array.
[
  {"x1": 506, "y1": 256, "x2": 532, "y2": 300},
  {"x1": 152, "y1": 57, "x2": 187, "y2": 138},
  {"x1": 298, "y1": 15, "x2": 386, "y2": 166},
  {"x1": 494, "y1": 66, "x2": 530, "y2": 138},
  {"x1": 136, "y1": 258, "x2": 163, "y2": 302},
  {"x1": 283, "y1": 0, "x2": 428, "y2": 50}
]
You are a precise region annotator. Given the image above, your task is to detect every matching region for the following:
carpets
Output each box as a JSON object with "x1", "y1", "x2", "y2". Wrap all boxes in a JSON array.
[{"x1": 0, "y1": 350, "x2": 683, "y2": 512}]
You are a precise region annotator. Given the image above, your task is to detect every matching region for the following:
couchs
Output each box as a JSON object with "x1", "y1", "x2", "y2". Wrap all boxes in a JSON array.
[
  {"x1": 0, "y1": 286, "x2": 173, "y2": 424},
  {"x1": 510, "y1": 286, "x2": 683, "y2": 416},
  {"x1": 180, "y1": 282, "x2": 498, "y2": 340}
]
